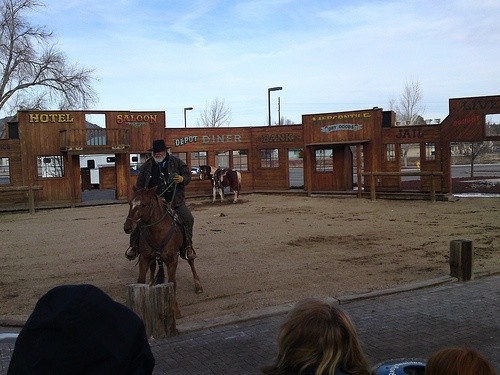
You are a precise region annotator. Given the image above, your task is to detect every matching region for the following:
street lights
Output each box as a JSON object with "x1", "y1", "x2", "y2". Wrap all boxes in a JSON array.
[
  {"x1": 184, "y1": 107, "x2": 192, "y2": 128},
  {"x1": 267, "y1": 87, "x2": 282, "y2": 126}
]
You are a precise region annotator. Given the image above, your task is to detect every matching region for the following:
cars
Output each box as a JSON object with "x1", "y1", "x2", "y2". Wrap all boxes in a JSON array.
[{"x1": 191, "y1": 168, "x2": 199, "y2": 174}]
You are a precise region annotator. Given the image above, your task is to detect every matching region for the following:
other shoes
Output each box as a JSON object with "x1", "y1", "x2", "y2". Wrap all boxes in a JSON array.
[
  {"x1": 181, "y1": 246, "x2": 197, "y2": 260},
  {"x1": 127, "y1": 248, "x2": 140, "y2": 258}
]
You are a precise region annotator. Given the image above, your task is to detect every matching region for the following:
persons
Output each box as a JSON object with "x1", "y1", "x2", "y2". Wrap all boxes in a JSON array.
[
  {"x1": 125, "y1": 139, "x2": 197, "y2": 261},
  {"x1": 425, "y1": 348, "x2": 497, "y2": 375},
  {"x1": 260, "y1": 297, "x2": 376, "y2": 375}
]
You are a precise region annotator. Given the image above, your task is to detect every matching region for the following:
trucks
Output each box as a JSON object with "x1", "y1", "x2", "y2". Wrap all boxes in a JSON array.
[{"x1": 41, "y1": 154, "x2": 141, "y2": 190}]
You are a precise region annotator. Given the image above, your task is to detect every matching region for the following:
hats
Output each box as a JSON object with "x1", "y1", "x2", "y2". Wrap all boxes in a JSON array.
[{"x1": 147, "y1": 139, "x2": 172, "y2": 153}]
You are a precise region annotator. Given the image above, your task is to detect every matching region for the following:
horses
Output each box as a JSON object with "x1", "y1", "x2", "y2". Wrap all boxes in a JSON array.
[
  {"x1": 123, "y1": 186, "x2": 203, "y2": 294},
  {"x1": 198, "y1": 165, "x2": 242, "y2": 204}
]
